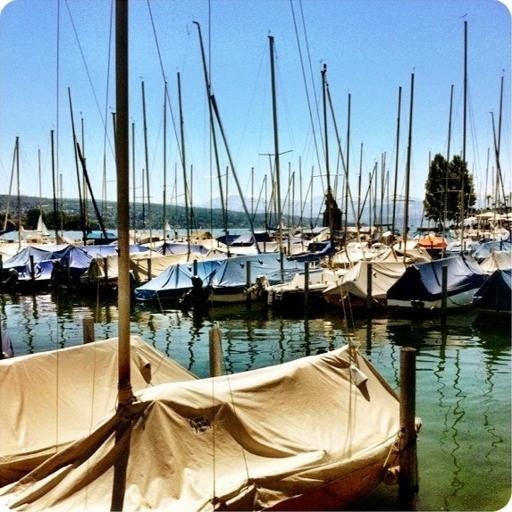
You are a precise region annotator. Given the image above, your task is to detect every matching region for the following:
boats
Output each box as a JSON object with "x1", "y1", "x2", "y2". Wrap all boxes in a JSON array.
[
  {"x1": 1, "y1": 332, "x2": 198, "y2": 480},
  {"x1": 1, "y1": 342, "x2": 421, "y2": 512}
]
